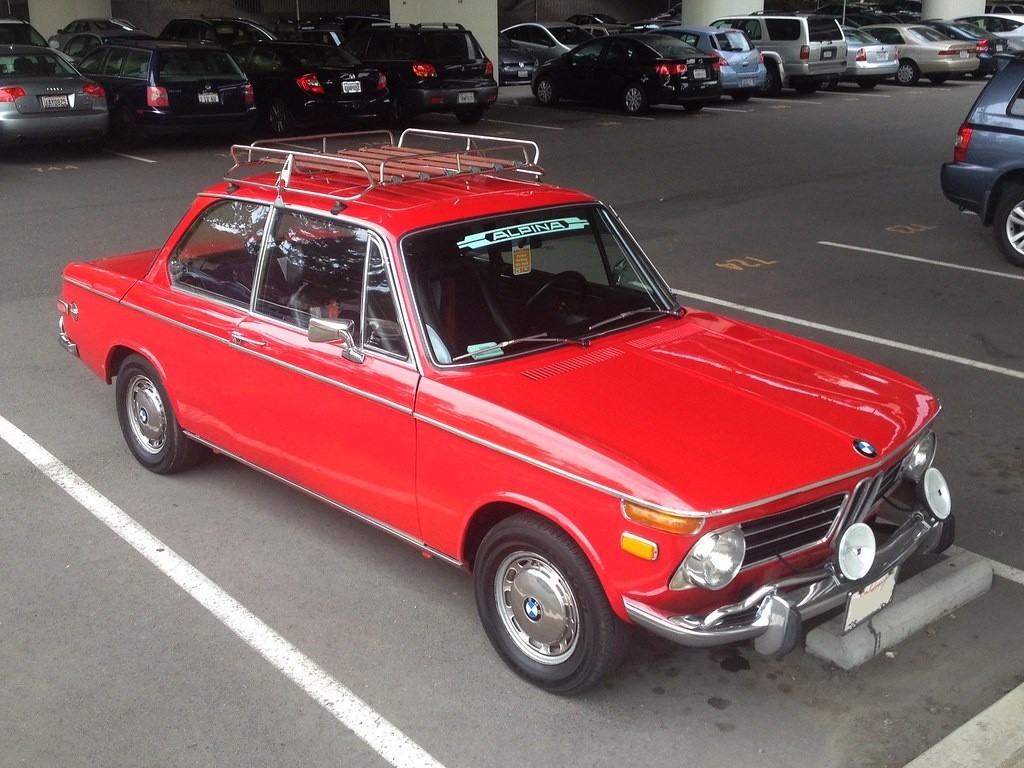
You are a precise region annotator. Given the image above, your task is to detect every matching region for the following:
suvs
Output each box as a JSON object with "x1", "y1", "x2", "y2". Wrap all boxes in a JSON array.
[
  {"x1": 157, "y1": 14, "x2": 279, "y2": 44},
  {"x1": 343, "y1": 22, "x2": 498, "y2": 129},
  {"x1": 706, "y1": 10, "x2": 848, "y2": 98}
]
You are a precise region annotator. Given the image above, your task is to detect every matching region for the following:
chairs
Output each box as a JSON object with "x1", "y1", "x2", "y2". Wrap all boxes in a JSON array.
[
  {"x1": 11, "y1": 59, "x2": 33, "y2": 74},
  {"x1": 413, "y1": 233, "x2": 517, "y2": 355},
  {"x1": 289, "y1": 242, "x2": 361, "y2": 329}
]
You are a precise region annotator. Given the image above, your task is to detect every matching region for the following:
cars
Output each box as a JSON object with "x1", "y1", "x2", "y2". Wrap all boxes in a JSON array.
[
  {"x1": 294, "y1": 14, "x2": 390, "y2": 29},
  {"x1": 985, "y1": 2, "x2": 1024, "y2": 14},
  {"x1": 0, "y1": 43, "x2": 108, "y2": 155},
  {"x1": 83, "y1": 45, "x2": 259, "y2": 154},
  {"x1": 0, "y1": 16, "x2": 50, "y2": 46},
  {"x1": 281, "y1": 29, "x2": 345, "y2": 48},
  {"x1": 841, "y1": 24, "x2": 900, "y2": 90},
  {"x1": 919, "y1": 18, "x2": 1012, "y2": 80},
  {"x1": 59, "y1": 32, "x2": 161, "y2": 58},
  {"x1": 863, "y1": 23, "x2": 980, "y2": 86},
  {"x1": 958, "y1": 12, "x2": 1024, "y2": 56},
  {"x1": 241, "y1": 41, "x2": 399, "y2": 137},
  {"x1": 530, "y1": 33, "x2": 723, "y2": 118},
  {"x1": 47, "y1": 17, "x2": 143, "y2": 31},
  {"x1": 55, "y1": 127, "x2": 954, "y2": 699},
  {"x1": 940, "y1": 54, "x2": 1024, "y2": 270},
  {"x1": 498, "y1": 0, "x2": 921, "y2": 87},
  {"x1": 643, "y1": 26, "x2": 767, "y2": 103}
]
